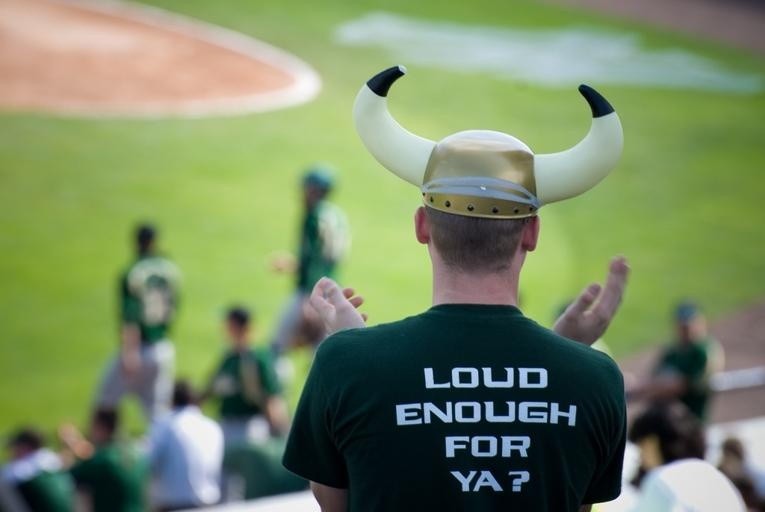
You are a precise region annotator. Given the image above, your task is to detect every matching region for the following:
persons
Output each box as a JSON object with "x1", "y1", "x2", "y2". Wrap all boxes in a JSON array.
[
  {"x1": 279, "y1": 61, "x2": 633, "y2": 511},
  {"x1": 222, "y1": 429, "x2": 310, "y2": 502},
  {"x1": 0, "y1": 424, "x2": 85, "y2": 512},
  {"x1": 127, "y1": 374, "x2": 226, "y2": 510},
  {"x1": 90, "y1": 217, "x2": 185, "y2": 428},
  {"x1": 185, "y1": 305, "x2": 289, "y2": 447},
  {"x1": 55, "y1": 403, "x2": 153, "y2": 511},
  {"x1": 261, "y1": 159, "x2": 351, "y2": 388}
]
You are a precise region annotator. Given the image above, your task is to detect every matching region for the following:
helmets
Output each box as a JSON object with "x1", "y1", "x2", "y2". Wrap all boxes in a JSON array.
[{"x1": 420, "y1": 127, "x2": 541, "y2": 221}]
